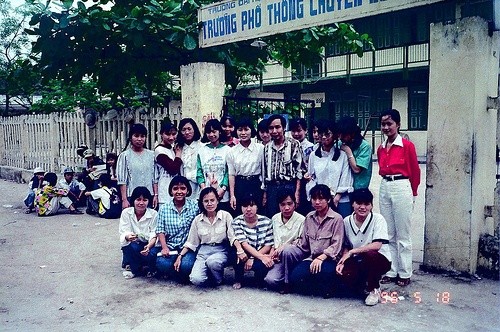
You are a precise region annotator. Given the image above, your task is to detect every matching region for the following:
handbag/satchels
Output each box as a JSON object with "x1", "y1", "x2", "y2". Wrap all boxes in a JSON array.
[{"x1": 306, "y1": 178, "x2": 318, "y2": 201}]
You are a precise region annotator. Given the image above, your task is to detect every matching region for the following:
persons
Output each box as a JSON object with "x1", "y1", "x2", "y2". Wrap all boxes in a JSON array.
[
  {"x1": 273, "y1": 184, "x2": 345, "y2": 299},
  {"x1": 174, "y1": 187, "x2": 248, "y2": 286},
  {"x1": 154, "y1": 176, "x2": 200, "y2": 280},
  {"x1": 264, "y1": 193, "x2": 306, "y2": 294},
  {"x1": 231, "y1": 193, "x2": 273, "y2": 290},
  {"x1": 118, "y1": 187, "x2": 159, "y2": 278},
  {"x1": 337, "y1": 187, "x2": 393, "y2": 306},
  {"x1": 154, "y1": 115, "x2": 373, "y2": 219},
  {"x1": 25, "y1": 146, "x2": 122, "y2": 219},
  {"x1": 376, "y1": 109, "x2": 420, "y2": 286},
  {"x1": 116, "y1": 124, "x2": 159, "y2": 279}
]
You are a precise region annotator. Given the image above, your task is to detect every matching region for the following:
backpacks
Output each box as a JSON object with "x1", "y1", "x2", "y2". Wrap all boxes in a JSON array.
[{"x1": 101, "y1": 188, "x2": 121, "y2": 219}]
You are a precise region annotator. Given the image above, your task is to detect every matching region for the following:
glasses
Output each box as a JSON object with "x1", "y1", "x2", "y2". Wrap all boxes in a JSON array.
[{"x1": 318, "y1": 132, "x2": 334, "y2": 138}]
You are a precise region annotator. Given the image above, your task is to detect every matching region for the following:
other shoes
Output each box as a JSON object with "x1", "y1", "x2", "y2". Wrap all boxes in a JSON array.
[
  {"x1": 380, "y1": 274, "x2": 399, "y2": 284},
  {"x1": 25, "y1": 207, "x2": 32, "y2": 214},
  {"x1": 70, "y1": 208, "x2": 84, "y2": 215},
  {"x1": 397, "y1": 277, "x2": 410, "y2": 286},
  {"x1": 123, "y1": 264, "x2": 135, "y2": 279},
  {"x1": 364, "y1": 288, "x2": 381, "y2": 305},
  {"x1": 86, "y1": 209, "x2": 92, "y2": 214}
]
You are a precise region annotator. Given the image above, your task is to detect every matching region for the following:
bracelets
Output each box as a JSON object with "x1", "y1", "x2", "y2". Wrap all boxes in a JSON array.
[
  {"x1": 317, "y1": 257, "x2": 324, "y2": 261},
  {"x1": 179, "y1": 254, "x2": 184, "y2": 256},
  {"x1": 250, "y1": 256, "x2": 255, "y2": 259},
  {"x1": 236, "y1": 251, "x2": 244, "y2": 255}
]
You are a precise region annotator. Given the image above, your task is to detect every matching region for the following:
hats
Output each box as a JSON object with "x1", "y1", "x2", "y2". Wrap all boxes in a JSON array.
[
  {"x1": 33, "y1": 167, "x2": 45, "y2": 175},
  {"x1": 83, "y1": 149, "x2": 95, "y2": 159},
  {"x1": 64, "y1": 167, "x2": 75, "y2": 175}
]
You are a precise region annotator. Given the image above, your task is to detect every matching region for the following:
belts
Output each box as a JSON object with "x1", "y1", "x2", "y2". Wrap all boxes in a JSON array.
[
  {"x1": 201, "y1": 242, "x2": 223, "y2": 247},
  {"x1": 267, "y1": 179, "x2": 293, "y2": 186},
  {"x1": 383, "y1": 174, "x2": 409, "y2": 181},
  {"x1": 236, "y1": 176, "x2": 259, "y2": 180}
]
